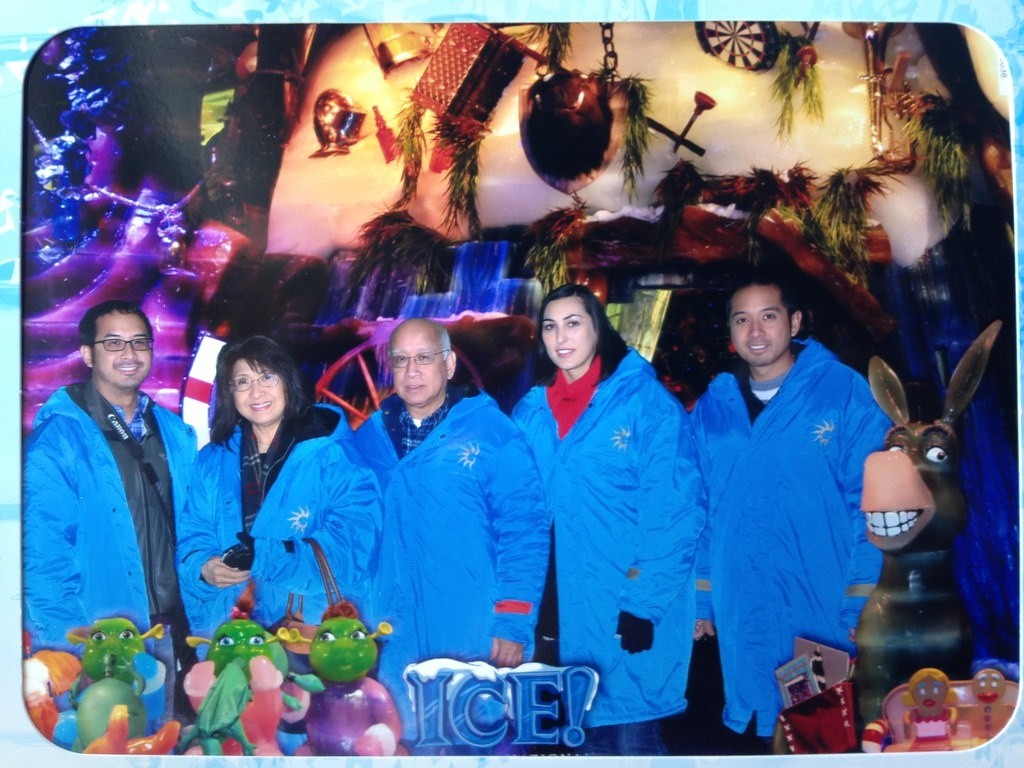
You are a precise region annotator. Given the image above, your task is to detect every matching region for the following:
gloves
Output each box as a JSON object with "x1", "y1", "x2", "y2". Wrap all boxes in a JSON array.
[
  {"x1": 221, "y1": 532, "x2": 255, "y2": 571},
  {"x1": 615, "y1": 610, "x2": 653, "y2": 654}
]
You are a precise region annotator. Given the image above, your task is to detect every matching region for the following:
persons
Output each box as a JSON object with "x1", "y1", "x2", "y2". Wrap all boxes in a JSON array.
[
  {"x1": 176, "y1": 333, "x2": 385, "y2": 755},
  {"x1": 505, "y1": 282, "x2": 710, "y2": 756},
  {"x1": 687, "y1": 267, "x2": 896, "y2": 753},
  {"x1": 21, "y1": 300, "x2": 199, "y2": 739},
  {"x1": 352, "y1": 316, "x2": 552, "y2": 754}
]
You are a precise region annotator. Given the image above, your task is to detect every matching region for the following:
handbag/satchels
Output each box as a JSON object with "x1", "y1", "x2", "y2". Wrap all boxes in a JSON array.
[{"x1": 266, "y1": 537, "x2": 388, "y2": 734}]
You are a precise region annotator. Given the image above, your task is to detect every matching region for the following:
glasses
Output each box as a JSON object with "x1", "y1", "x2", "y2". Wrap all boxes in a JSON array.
[
  {"x1": 90, "y1": 338, "x2": 154, "y2": 352},
  {"x1": 229, "y1": 372, "x2": 280, "y2": 392},
  {"x1": 389, "y1": 349, "x2": 450, "y2": 368}
]
